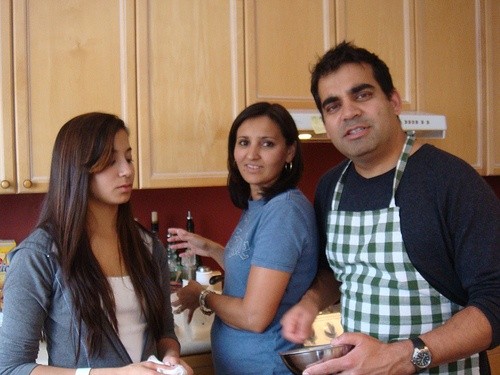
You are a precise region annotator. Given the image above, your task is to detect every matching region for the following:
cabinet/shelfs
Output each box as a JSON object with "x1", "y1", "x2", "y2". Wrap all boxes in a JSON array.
[{"x1": 0, "y1": 0, "x2": 500, "y2": 195}]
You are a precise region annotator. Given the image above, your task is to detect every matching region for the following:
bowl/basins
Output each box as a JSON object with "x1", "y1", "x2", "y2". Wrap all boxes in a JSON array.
[{"x1": 280, "y1": 344, "x2": 354, "y2": 375}]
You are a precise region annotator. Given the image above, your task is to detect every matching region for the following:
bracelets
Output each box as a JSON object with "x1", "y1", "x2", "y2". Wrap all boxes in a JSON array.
[{"x1": 199, "y1": 289, "x2": 215, "y2": 315}]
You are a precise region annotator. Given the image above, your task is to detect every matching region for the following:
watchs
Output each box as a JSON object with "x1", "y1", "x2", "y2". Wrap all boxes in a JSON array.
[{"x1": 409, "y1": 337, "x2": 432, "y2": 373}]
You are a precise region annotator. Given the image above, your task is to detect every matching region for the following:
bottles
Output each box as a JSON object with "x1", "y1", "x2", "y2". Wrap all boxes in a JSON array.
[
  {"x1": 180, "y1": 211, "x2": 200, "y2": 266},
  {"x1": 149, "y1": 211, "x2": 159, "y2": 236},
  {"x1": 165, "y1": 233, "x2": 177, "y2": 282},
  {"x1": 195, "y1": 266, "x2": 214, "y2": 292}
]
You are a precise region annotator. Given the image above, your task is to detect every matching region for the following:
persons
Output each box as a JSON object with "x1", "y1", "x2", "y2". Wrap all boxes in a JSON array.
[
  {"x1": 280, "y1": 40, "x2": 500, "y2": 375},
  {"x1": 0, "y1": 112, "x2": 193, "y2": 375},
  {"x1": 167, "y1": 101, "x2": 317, "y2": 375}
]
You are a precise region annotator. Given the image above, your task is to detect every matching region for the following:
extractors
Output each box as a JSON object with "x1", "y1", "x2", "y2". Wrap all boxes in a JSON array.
[{"x1": 288, "y1": 108, "x2": 448, "y2": 141}]
